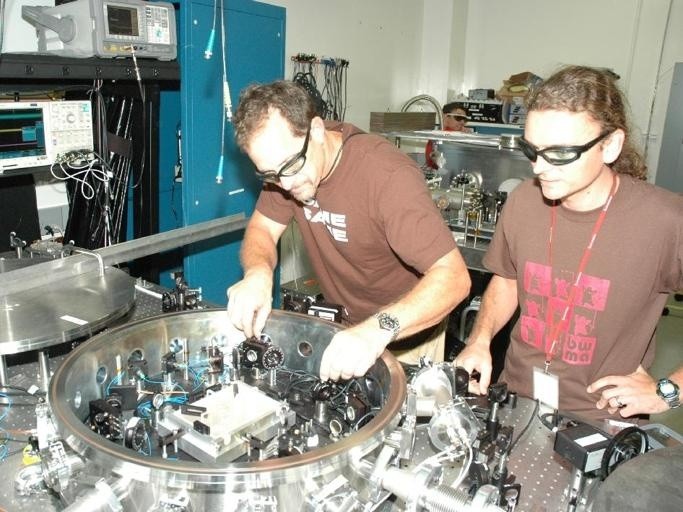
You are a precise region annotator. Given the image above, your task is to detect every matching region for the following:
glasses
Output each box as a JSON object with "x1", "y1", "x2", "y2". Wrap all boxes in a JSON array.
[
  {"x1": 446, "y1": 111, "x2": 468, "y2": 123},
  {"x1": 255, "y1": 125, "x2": 312, "y2": 183},
  {"x1": 518, "y1": 129, "x2": 612, "y2": 165}
]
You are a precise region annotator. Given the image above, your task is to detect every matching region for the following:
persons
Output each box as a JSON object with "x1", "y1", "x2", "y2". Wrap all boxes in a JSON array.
[
  {"x1": 227, "y1": 80, "x2": 472, "y2": 382},
  {"x1": 449, "y1": 66, "x2": 683, "y2": 435},
  {"x1": 443, "y1": 104, "x2": 472, "y2": 132}
]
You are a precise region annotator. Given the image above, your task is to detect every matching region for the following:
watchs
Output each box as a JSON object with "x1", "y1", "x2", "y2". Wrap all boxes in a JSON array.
[
  {"x1": 656, "y1": 379, "x2": 681, "y2": 408},
  {"x1": 373, "y1": 312, "x2": 400, "y2": 342}
]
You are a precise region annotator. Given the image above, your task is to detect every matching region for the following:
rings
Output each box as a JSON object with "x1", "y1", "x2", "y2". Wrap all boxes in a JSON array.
[{"x1": 615, "y1": 397, "x2": 623, "y2": 406}]
